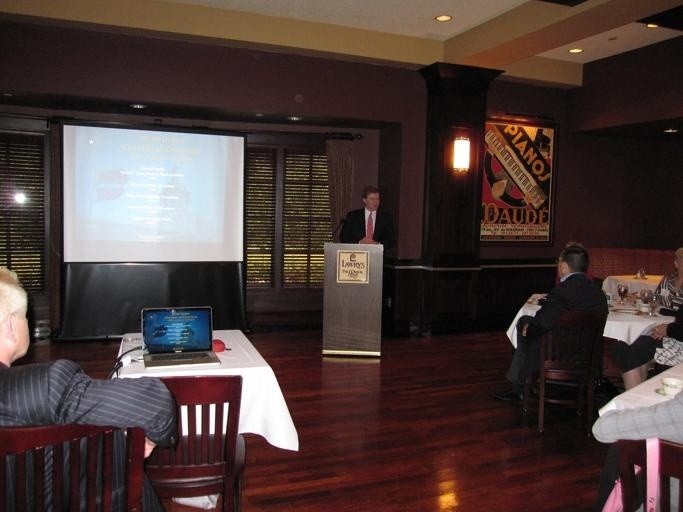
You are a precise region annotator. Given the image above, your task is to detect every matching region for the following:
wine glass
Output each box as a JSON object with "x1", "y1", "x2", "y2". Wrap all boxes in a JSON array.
[
  {"x1": 640, "y1": 289, "x2": 649, "y2": 304},
  {"x1": 648, "y1": 293, "x2": 658, "y2": 317},
  {"x1": 618, "y1": 283, "x2": 628, "y2": 305}
]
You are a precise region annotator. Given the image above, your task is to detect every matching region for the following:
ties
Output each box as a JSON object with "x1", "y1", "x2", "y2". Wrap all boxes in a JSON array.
[{"x1": 366, "y1": 212, "x2": 374, "y2": 240}]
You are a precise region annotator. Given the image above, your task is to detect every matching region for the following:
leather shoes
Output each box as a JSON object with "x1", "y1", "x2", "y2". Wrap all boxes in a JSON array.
[{"x1": 494, "y1": 390, "x2": 524, "y2": 405}]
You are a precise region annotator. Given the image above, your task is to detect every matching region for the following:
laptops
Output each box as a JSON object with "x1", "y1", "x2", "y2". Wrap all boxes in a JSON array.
[{"x1": 139, "y1": 304, "x2": 222, "y2": 370}]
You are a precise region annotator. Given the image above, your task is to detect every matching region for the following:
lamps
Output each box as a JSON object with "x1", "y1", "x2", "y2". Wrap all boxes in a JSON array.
[{"x1": 452, "y1": 137, "x2": 470, "y2": 177}]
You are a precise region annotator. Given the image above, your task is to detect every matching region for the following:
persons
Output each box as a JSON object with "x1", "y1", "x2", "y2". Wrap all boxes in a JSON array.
[
  {"x1": 338, "y1": 185, "x2": 397, "y2": 251},
  {"x1": 616, "y1": 245, "x2": 683, "y2": 391},
  {"x1": 0, "y1": 266, "x2": 181, "y2": 512},
  {"x1": 487, "y1": 240, "x2": 610, "y2": 406}
]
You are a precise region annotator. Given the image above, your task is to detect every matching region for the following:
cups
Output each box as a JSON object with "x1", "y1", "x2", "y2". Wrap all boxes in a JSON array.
[
  {"x1": 638, "y1": 302, "x2": 650, "y2": 313},
  {"x1": 660, "y1": 378, "x2": 682, "y2": 395}
]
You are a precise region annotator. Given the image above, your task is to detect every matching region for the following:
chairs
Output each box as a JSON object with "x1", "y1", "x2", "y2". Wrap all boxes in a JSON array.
[
  {"x1": 523, "y1": 309, "x2": 608, "y2": 433},
  {"x1": 146, "y1": 376, "x2": 246, "y2": 512},
  {"x1": 0, "y1": 423, "x2": 146, "y2": 511},
  {"x1": 610, "y1": 438, "x2": 683, "y2": 512}
]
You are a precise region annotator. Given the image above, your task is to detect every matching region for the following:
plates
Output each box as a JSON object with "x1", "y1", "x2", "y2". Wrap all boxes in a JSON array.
[{"x1": 654, "y1": 387, "x2": 674, "y2": 399}]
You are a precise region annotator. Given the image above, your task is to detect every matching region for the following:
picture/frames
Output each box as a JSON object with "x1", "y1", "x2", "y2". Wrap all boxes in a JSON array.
[{"x1": 479, "y1": 119, "x2": 556, "y2": 246}]
